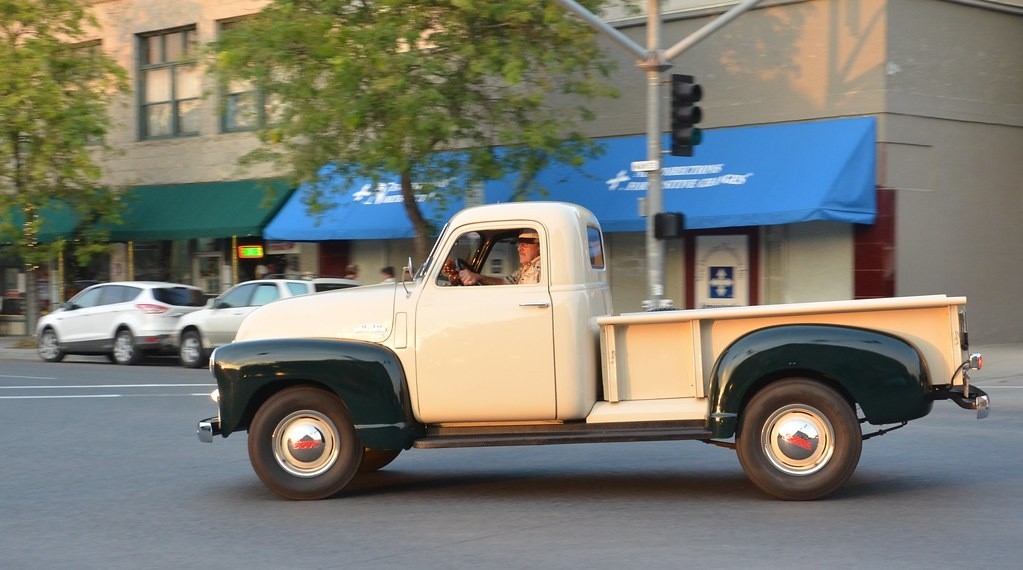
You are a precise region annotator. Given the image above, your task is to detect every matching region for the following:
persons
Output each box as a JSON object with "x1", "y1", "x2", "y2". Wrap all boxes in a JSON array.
[
  {"x1": 345, "y1": 264, "x2": 414, "y2": 283},
  {"x1": 256, "y1": 266, "x2": 270, "y2": 280},
  {"x1": 458, "y1": 228, "x2": 541, "y2": 285}
]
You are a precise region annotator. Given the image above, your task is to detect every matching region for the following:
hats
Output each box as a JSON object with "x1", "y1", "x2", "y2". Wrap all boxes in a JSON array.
[
  {"x1": 518, "y1": 227, "x2": 539, "y2": 238},
  {"x1": 256, "y1": 265, "x2": 268, "y2": 274},
  {"x1": 346, "y1": 264, "x2": 358, "y2": 273},
  {"x1": 379, "y1": 266, "x2": 395, "y2": 275}
]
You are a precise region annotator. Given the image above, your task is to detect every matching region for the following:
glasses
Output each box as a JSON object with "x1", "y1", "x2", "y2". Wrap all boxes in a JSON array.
[{"x1": 515, "y1": 240, "x2": 538, "y2": 248}]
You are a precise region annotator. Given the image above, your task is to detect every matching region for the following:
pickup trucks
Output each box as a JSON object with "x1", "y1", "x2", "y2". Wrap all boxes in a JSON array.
[{"x1": 201, "y1": 200, "x2": 990, "y2": 496}]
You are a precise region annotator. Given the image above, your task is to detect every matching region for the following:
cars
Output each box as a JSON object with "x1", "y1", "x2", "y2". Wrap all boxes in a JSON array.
[{"x1": 37, "y1": 281, "x2": 208, "y2": 366}]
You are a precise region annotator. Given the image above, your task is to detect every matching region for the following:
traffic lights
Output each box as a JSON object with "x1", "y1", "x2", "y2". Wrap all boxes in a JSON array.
[
  {"x1": 668, "y1": 73, "x2": 703, "y2": 157},
  {"x1": 653, "y1": 211, "x2": 683, "y2": 239}
]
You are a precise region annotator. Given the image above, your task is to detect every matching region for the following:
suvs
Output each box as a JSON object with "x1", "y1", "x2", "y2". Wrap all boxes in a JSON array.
[{"x1": 173, "y1": 275, "x2": 363, "y2": 368}]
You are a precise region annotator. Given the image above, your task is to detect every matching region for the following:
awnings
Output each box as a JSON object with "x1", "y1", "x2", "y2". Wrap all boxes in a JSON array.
[
  {"x1": 85, "y1": 175, "x2": 297, "y2": 241},
  {"x1": 261, "y1": 115, "x2": 879, "y2": 240},
  {"x1": 0, "y1": 188, "x2": 115, "y2": 243}
]
